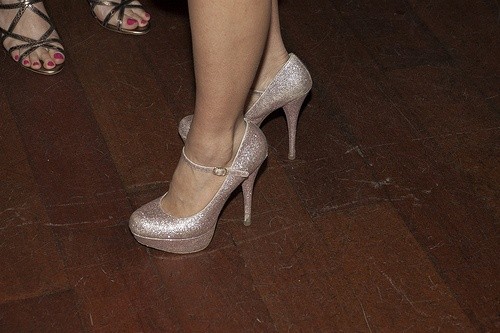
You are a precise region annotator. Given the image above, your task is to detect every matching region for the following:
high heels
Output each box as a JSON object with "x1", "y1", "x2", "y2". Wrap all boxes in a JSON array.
[
  {"x1": 129, "y1": 118, "x2": 268, "y2": 254},
  {"x1": 179, "y1": 53, "x2": 313, "y2": 160}
]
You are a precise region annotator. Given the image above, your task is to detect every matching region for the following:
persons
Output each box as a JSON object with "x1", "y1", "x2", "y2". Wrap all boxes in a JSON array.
[
  {"x1": 129, "y1": 0, "x2": 312, "y2": 254},
  {"x1": 0, "y1": 0, "x2": 152, "y2": 75}
]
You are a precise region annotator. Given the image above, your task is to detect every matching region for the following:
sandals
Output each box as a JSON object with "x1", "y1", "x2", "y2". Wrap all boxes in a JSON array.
[
  {"x1": 0, "y1": 0, "x2": 67, "y2": 75},
  {"x1": 87, "y1": 0, "x2": 152, "y2": 35}
]
